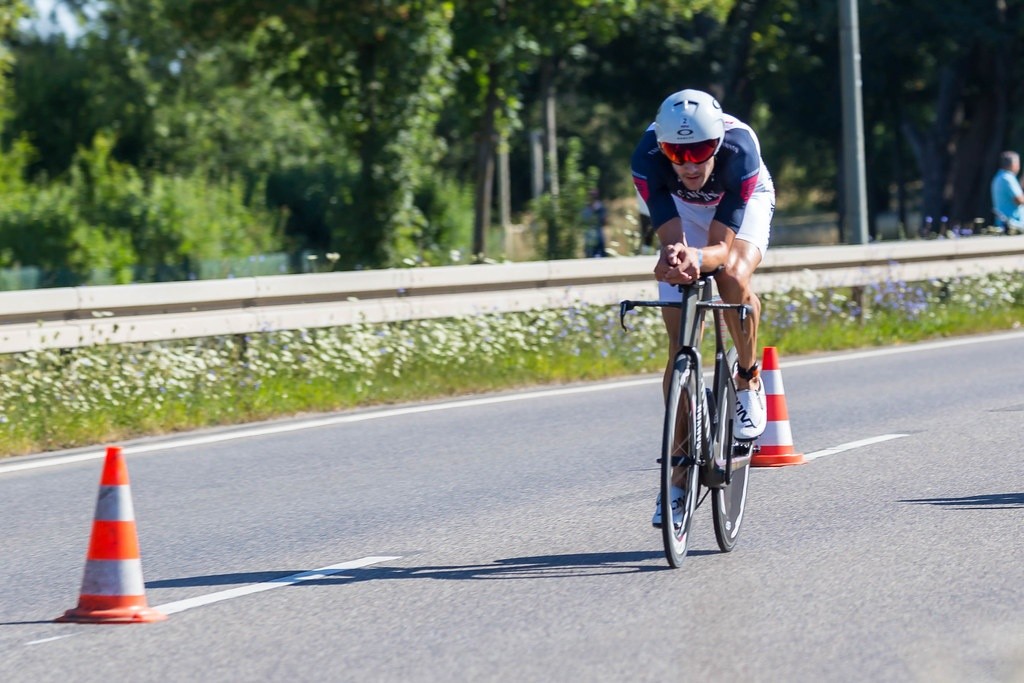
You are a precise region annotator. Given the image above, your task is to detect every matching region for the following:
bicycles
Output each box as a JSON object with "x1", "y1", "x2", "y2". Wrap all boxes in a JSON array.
[{"x1": 619, "y1": 266, "x2": 758, "y2": 568}]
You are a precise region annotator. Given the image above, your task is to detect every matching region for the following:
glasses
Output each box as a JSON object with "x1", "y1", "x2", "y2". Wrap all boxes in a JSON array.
[{"x1": 660, "y1": 138, "x2": 718, "y2": 165}]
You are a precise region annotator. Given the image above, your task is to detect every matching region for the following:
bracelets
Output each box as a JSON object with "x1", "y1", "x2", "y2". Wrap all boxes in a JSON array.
[{"x1": 698, "y1": 249, "x2": 702, "y2": 267}]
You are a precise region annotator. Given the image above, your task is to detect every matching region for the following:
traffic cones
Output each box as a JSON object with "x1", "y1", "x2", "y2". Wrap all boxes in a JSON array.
[
  {"x1": 750, "y1": 347, "x2": 804, "y2": 467},
  {"x1": 52, "y1": 446, "x2": 165, "y2": 623}
]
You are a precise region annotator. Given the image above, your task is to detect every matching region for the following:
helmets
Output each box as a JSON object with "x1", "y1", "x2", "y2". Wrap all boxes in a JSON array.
[{"x1": 655, "y1": 89, "x2": 725, "y2": 155}]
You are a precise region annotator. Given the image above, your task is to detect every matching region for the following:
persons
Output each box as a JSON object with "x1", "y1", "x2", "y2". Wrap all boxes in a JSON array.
[
  {"x1": 991, "y1": 151, "x2": 1024, "y2": 233},
  {"x1": 629, "y1": 90, "x2": 776, "y2": 530}
]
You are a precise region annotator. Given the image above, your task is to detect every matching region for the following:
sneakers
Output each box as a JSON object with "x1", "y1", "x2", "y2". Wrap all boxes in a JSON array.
[
  {"x1": 732, "y1": 376, "x2": 767, "y2": 442},
  {"x1": 652, "y1": 485, "x2": 686, "y2": 530}
]
